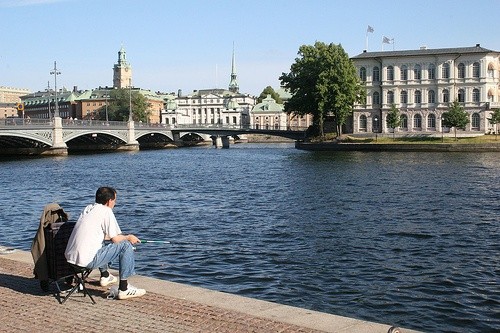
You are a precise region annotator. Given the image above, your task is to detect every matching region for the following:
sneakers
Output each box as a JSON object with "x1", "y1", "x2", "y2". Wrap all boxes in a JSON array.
[
  {"x1": 100, "y1": 273, "x2": 117, "y2": 286},
  {"x1": 117, "y1": 284, "x2": 146, "y2": 299}
]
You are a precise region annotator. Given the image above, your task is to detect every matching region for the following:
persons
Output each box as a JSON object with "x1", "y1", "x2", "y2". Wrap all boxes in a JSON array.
[
  {"x1": 151, "y1": 120, "x2": 178, "y2": 128},
  {"x1": 27, "y1": 116, "x2": 30, "y2": 122},
  {"x1": 65, "y1": 186, "x2": 146, "y2": 299},
  {"x1": 65, "y1": 116, "x2": 110, "y2": 126}
]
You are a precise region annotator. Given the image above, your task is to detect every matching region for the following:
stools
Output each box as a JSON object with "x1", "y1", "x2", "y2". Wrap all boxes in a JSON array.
[{"x1": 49, "y1": 262, "x2": 97, "y2": 305}]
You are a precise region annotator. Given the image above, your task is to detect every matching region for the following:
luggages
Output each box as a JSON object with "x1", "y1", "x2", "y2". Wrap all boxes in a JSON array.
[{"x1": 40, "y1": 203, "x2": 78, "y2": 291}]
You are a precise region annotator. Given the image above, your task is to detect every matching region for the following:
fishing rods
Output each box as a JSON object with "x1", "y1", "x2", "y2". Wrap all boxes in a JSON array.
[{"x1": 132, "y1": 239, "x2": 212, "y2": 251}]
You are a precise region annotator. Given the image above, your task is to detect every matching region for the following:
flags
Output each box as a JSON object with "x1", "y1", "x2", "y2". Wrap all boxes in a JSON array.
[
  {"x1": 383, "y1": 36, "x2": 390, "y2": 44},
  {"x1": 17, "y1": 103, "x2": 24, "y2": 110},
  {"x1": 367, "y1": 25, "x2": 375, "y2": 33}
]
